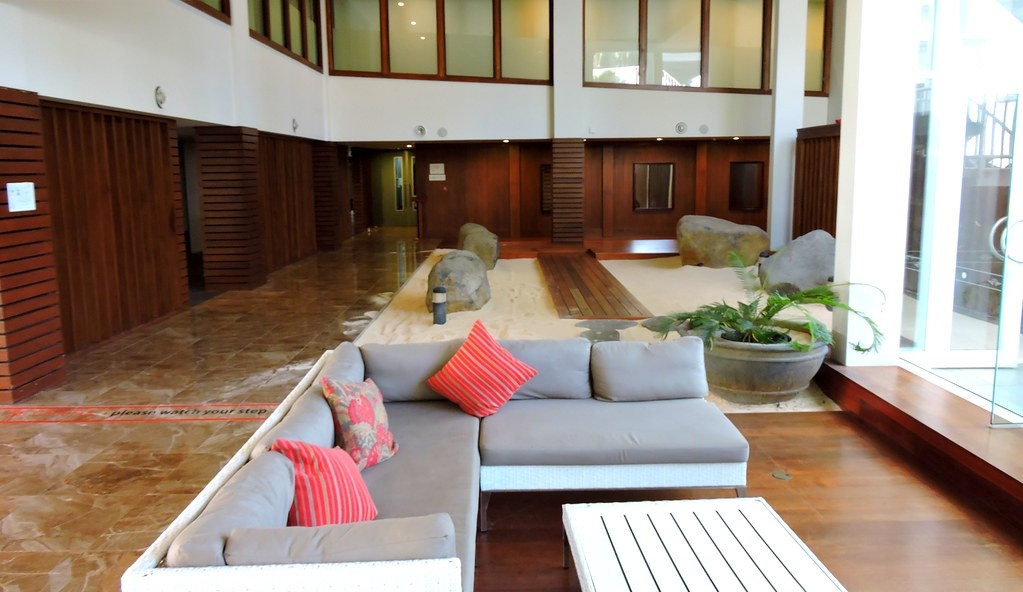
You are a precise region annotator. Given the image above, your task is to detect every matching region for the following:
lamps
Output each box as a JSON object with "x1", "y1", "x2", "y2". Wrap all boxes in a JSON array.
[
  {"x1": 416, "y1": 126, "x2": 426, "y2": 137},
  {"x1": 675, "y1": 122, "x2": 687, "y2": 134},
  {"x1": 758, "y1": 251, "x2": 769, "y2": 264},
  {"x1": 293, "y1": 118, "x2": 298, "y2": 132},
  {"x1": 431, "y1": 287, "x2": 447, "y2": 304},
  {"x1": 156, "y1": 86, "x2": 166, "y2": 108}
]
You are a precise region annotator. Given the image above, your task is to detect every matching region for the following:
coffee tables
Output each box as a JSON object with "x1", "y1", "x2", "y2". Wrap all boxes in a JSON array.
[{"x1": 561, "y1": 498, "x2": 849, "y2": 592}]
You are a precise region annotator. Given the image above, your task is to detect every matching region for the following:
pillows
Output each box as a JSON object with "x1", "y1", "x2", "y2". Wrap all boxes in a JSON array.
[
  {"x1": 322, "y1": 375, "x2": 399, "y2": 472},
  {"x1": 270, "y1": 439, "x2": 378, "y2": 527},
  {"x1": 424, "y1": 321, "x2": 539, "y2": 418}
]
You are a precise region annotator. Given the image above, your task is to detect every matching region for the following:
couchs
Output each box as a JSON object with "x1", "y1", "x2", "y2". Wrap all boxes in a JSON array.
[{"x1": 137, "y1": 336, "x2": 751, "y2": 592}]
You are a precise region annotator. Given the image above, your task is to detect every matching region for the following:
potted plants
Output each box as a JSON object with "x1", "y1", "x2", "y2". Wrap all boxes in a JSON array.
[{"x1": 655, "y1": 250, "x2": 886, "y2": 392}]
